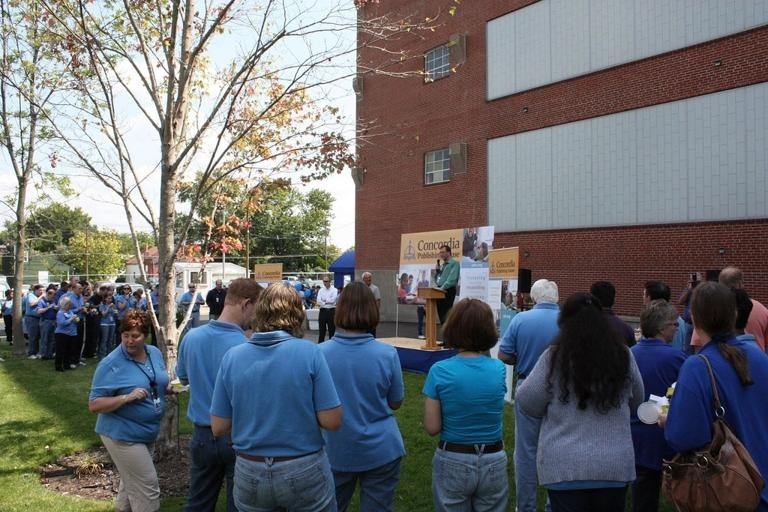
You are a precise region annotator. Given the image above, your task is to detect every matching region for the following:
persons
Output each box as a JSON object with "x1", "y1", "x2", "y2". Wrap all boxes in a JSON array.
[
  {"x1": 434, "y1": 245, "x2": 460, "y2": 349},
  {"x1": 209, "y1": 282, "x2": 342, "y2": 510},
  {"x1": 89, "y1": 309, "x2": 179, "y2": 509},
  {"x1": 473, "y1": 241, "x2": 488, "y2": 261},
  {"x1": 417, "y1": 270, "x2": 428, "y2": 287},
  {"x1": 496, "y1": 264, "x2": 768, "y2": 512},
  {"x1": 205, "y1": 279, "x2": 228, "y2": 321},
  {"x1": 180, "y1": 283, "x2": 205, "y2": 334},
  {"x1": 1, "y1": 272, "x2": 159, "y2": 373},
  {"x1": 404, "y1": 275, "x2": 413, "y2": 293},
  {"x1": 175, "y1": 277, "x2": 265, "y2": 510},
  {"x1": 398, "y1": 273, "x2": 417, "y2": 305},
  {"x1": 292, "y1": 273, "x2": 381, "y2": 344},
  {"x1": 421, "y1": 297, "x2": 510, "y2": 511},
  {"x1": 317, "y1": 281, "x2": 406, "y2": 510},
  {"x1": 416, "y1": 305, "x2": 425, "y2": 339},
  {"x1": 463, "y1": 228, "x2": 477, "y2": 256}
]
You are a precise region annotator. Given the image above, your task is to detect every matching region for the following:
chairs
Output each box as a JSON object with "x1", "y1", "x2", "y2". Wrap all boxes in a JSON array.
[
  {"x1": 28, "y1": 353, "x2": 43, "y2": 360},
  {"x1": 418, "y1": 334, "x2": 427, "y2": 341},
  {"x1": 55, "y1": 354, "x2": 98, "y2": 371}
]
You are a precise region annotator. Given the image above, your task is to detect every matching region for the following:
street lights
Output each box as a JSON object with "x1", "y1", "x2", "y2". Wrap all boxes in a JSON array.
[{"x1": 657, "y1": 353, "x2": 766, "y2": 512}]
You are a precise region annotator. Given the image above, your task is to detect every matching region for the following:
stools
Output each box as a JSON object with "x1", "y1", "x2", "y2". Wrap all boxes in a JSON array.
[
  {"x1": 236, "y1": 452, "x2": 312, "y2": 465},
  {"x1": 434, "y1": 440, "x2": 506, "y2": 458}
]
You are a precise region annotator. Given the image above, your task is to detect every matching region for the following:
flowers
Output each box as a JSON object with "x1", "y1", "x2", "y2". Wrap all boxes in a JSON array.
[{"x1": 437, "y1": 259, "x2": 440, "y2": 266}]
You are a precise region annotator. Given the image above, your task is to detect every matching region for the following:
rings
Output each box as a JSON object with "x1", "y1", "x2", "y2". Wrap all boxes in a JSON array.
[{"x1": 140, "y1": 390, "x2": 143, "y2": 393}]
